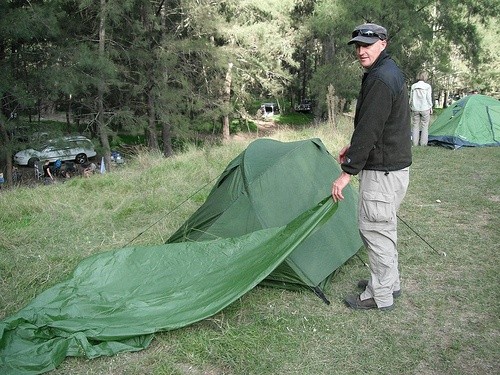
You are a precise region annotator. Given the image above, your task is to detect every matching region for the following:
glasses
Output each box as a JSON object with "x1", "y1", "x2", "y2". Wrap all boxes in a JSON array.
[{"x1": 351, "y1": 29, "x2": 384, "y2": 41}]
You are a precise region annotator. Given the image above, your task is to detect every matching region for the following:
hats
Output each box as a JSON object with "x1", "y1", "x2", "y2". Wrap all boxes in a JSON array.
[{"x1": 347, "y1": 23, "x2": 387, "y2": 45}]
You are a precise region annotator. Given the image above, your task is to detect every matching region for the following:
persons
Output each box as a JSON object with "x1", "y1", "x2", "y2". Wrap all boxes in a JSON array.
[
  {"x1": 43, "y1": 160, "x2": 56, "y2": 185},
  {"x1": 331, "y1": 23, "x2": 412, "y2": 311},
  {"x1": 83, "y1": 162, "x2": 96, "y2": 175},
  {"x1": 410, "y1": 70, "x2": 433, "y2": 147}
]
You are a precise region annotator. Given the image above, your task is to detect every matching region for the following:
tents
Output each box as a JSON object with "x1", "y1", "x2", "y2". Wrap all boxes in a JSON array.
[
  {"x1": 411, "y1": 94, "x2": 500, "y2": 150},
  {"x1": 163, "y1": 138, "x2": 363, "y2": 307}
]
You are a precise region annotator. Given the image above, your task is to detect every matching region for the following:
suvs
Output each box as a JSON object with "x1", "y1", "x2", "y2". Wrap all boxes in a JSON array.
[
  {"x1": 297, "y1": 98, "x2": 318, "y2": 113},
  {"x1": 13, "y1": 135, "x2": 97, "y2": 168}
]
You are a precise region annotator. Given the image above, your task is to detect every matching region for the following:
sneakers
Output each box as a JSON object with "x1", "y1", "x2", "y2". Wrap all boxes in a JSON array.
[
  {"x1": 358, "y1": 280, "x2": 401, "y2": 297},
  {"x1": 345, "y1": 295, "x2": 394, "y2": 311}
]
